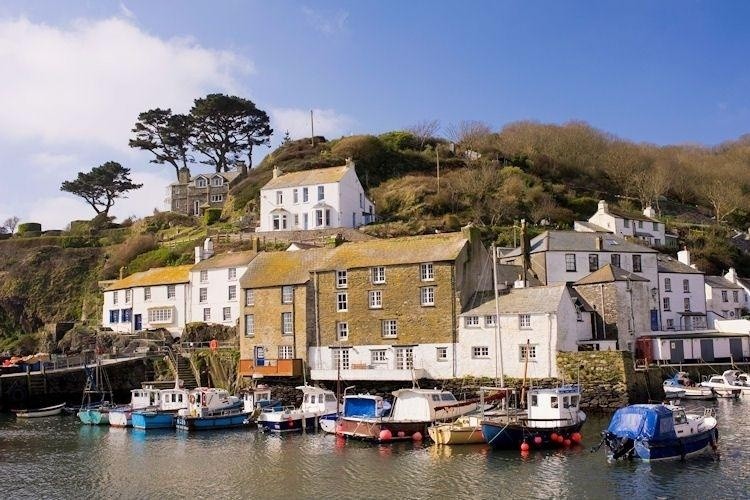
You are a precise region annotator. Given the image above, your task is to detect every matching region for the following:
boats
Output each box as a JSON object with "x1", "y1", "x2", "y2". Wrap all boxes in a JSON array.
[
  {"x1": 656, "y1": 365, "x2": 750, "y2": 404},
  {"x1": 596, "y1": 404, "x2": 724, "y2": 469},
  {"x1": 6, "y1": 401, "x2": 68, "y2": 423}
]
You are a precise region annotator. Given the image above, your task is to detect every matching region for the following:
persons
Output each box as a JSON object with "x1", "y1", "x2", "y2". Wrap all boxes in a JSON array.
[
  {"x1": 188, "y1": 339, "x2": 195, "y2": 353},
  {"x1": 237, "y1": 384, "x2": 253, "y2": 399}
]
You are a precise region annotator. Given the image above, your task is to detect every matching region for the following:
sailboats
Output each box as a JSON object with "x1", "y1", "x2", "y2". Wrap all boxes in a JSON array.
[{"x1": 424, "y1": 238, "x2": 524, "y2": 447}]
